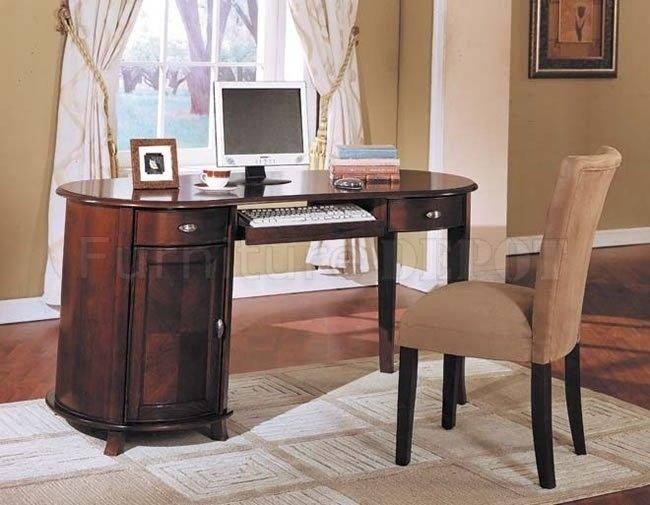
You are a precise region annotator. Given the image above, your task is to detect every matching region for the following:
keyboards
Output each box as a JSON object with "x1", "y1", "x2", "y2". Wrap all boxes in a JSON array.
[{"x1": 237, "y1": 203, "x2": 376, "y2": 228}]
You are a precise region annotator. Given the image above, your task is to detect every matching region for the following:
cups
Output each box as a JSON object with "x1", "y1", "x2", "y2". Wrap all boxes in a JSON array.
[{"x1": 200, "y1": 169, "x2": 231, "y2": 188}]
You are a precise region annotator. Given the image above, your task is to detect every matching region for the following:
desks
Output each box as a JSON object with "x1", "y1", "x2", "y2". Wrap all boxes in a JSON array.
[{"x1": 46, "y1": 170, "x2": 478, "y2": 456}]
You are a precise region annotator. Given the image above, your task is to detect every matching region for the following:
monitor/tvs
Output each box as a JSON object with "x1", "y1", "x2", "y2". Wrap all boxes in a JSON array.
[{"x1": 214, "y1": 81, "x2": 310, "y2": 186}]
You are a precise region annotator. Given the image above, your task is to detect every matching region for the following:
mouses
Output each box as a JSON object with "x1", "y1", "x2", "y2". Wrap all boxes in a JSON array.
[{"x1": 334, "y1": 177, "x2": 364, "y2": 190}]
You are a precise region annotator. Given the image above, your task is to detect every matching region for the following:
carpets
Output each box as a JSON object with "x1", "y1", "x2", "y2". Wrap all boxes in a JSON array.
[{"x1": 0, "y1": 350, "x2": 650, "y2": 504}]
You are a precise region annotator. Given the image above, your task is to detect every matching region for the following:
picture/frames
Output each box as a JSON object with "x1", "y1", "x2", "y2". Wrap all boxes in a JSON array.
[
  {"x1": 528, "y1": 0, "x2": 619, "y2": 78},
  {"x1": 131, "y1": 138, "x2": 180, "y2": 189}
]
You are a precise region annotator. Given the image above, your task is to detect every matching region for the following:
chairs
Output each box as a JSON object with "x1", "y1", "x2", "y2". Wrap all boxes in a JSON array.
[{"x1": 396, "y1": 145, "x2": 622, "y2": 489}]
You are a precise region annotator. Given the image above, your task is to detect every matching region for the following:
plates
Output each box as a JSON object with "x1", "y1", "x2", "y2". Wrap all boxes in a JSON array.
[{"x1": 192, "y1": 183, "x2": 241, "y2": 195}]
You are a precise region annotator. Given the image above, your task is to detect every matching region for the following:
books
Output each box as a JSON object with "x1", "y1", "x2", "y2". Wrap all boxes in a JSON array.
[{"x1": 330, "y1": 144, "x2": 401, "y2": 181}]
